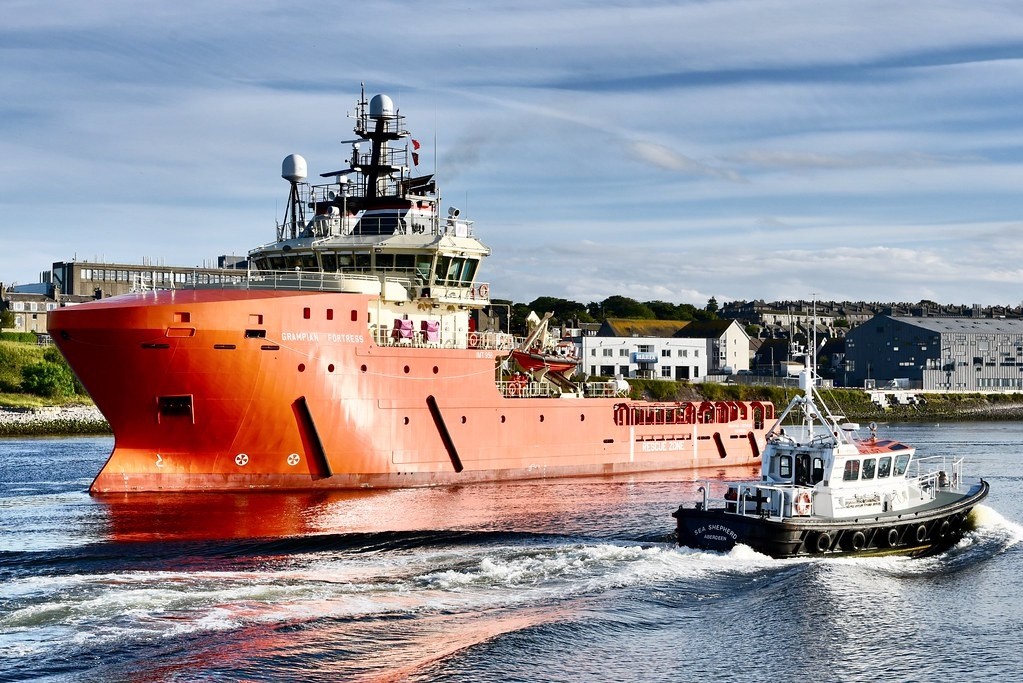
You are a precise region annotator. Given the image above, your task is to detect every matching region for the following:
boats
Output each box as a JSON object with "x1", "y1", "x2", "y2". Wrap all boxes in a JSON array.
[
  {"x1": 668, "y1": 287, "x2": 990, "y2": 558},
  {"x1": 510, "y1": 305, "x2": 581, "y2": 375},
  {"x1": 43, "y1": 78, "x2": 793, "y2": 550}
]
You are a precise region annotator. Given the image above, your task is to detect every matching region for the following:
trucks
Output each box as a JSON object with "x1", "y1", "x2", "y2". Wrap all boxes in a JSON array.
[{"x1": 884, "y1": 378, "x2": 910, "y2": 390}]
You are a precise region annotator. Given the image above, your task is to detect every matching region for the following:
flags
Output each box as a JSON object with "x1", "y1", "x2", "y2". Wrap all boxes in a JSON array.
[
  {"x1": 412, "y1": 152, "x2": 419, "y2": 166},
  {"x1": 412, "y1": 139, "x2": 420, "y2": 149}
]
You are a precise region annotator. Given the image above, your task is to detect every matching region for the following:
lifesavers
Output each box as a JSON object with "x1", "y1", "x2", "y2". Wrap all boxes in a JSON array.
[
  {"x1": 886, "y1": 528, "x2": 899, "y2": 547},
  {"x1": 851, "y1": 531, "x2": 866, "y2": 551},
  {"x1": 480, "y1": 285, "x2": 488, "y2": 297},
  {"x1": 939, "y1": 520, "x2": 949, "y2": 539},
  {"x1": 816, "y1": 532, "x2": 831, "y2": 552},
  {"x1": 915, "y1": 526, "x2": 927, "y2": 542},
  {"x1": 796, "y1": 494, "x2": 811, "y2": 516},
  {"x1": 958, "y1": 516, "x2": 968, "y2": 530},
  {"x1": 469, "y1": 335, "x2": 478, "y2": 346},
  {"x1": 509, "y1": 384, "x2": 516, "y2": 394}
]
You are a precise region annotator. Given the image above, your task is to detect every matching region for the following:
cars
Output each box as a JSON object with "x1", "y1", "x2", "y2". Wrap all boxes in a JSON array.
[
  {"x1": 737, "y1": 369, "x2": 753, "y2": 376},
  {"x1": 708, "y1": 367, "x2": 732, "y2": 375}
]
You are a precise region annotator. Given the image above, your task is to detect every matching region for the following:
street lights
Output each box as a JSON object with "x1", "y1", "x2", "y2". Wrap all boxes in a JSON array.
[{"x1": 769, "y1": 347, "x2": 775, "y2": 380}]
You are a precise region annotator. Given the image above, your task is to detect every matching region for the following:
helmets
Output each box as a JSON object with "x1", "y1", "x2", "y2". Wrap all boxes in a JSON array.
[
  {"x1": 515, "y1": 371, "x2": 519, "y2": 375},
  {"x1": 524, "y1": 371, "x2": 529, "y2": 374},
  {"x1": 530, "y1": 367, "x2": 534, "y2": 371}
]
